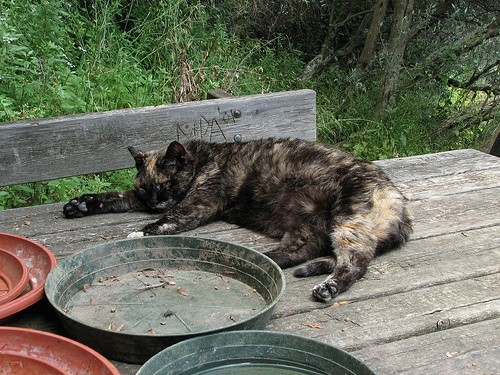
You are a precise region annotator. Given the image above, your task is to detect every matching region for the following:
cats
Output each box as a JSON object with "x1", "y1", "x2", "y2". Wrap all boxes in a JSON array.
[{"x1": 61, "y1": 138, "x2": 412, "y2": 302}]
